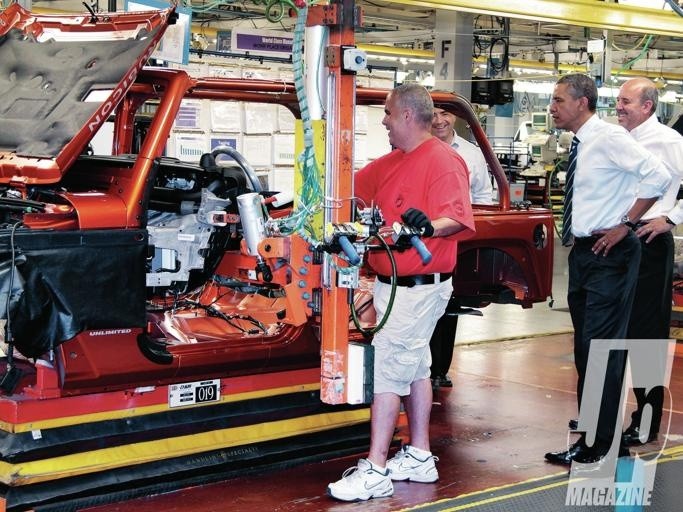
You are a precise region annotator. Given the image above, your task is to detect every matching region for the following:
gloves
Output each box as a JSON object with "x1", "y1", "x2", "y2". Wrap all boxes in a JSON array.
[{"x1": 401, "y1": 208, "x2": 434, "y2": 237}]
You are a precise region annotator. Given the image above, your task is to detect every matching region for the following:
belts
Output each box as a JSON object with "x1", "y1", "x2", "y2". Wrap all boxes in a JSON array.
[
  {"x1": 377, "y1": 273, "x2": 453, "y2": 290},
  {"x1": 574, "y1": 235, "x2": 603, "y2": 243},
  {"x1": 636, "y1": 224, "x2": 644, "y2": 227}
]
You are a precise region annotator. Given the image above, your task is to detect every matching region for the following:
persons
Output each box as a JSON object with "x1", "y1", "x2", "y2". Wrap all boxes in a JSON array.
[
  {"x1": 327, "y1": 84, "x2": 493, "y2": 501},
  {"x1": 545, "y1": 74, "x2": 683, "y2": 476}
]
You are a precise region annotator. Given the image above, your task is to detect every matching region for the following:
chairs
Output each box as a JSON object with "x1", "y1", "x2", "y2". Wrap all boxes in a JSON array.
[{"x1": 209, "y1": 149, "x2": 291, "y2": 218}]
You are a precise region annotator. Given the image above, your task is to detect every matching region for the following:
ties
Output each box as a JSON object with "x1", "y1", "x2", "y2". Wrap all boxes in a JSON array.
[{"x1": 562, "y1": 136, "x2": 580, "y2": 247}]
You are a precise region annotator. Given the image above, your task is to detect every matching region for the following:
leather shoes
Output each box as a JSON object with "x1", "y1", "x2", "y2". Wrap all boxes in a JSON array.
[
  {"x1": 431, "y1": 375, "x2": 453, "y2": 392},
  {"x1": 545, "y1": 420, "x2": 602, "y2": 465},
  {"x1": 617, "y1": 422, "x2": 657, "y2": 447}
]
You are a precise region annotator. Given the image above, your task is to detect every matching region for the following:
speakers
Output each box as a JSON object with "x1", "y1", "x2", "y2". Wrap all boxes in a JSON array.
[{"x1": 471, "y1": 76, "x2": 514, "y2": 106}]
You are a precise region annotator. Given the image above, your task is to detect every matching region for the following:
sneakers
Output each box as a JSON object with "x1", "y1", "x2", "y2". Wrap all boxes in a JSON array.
[{"x1": 326, "y1": 443, "x2": 439, "y2": 502}]
